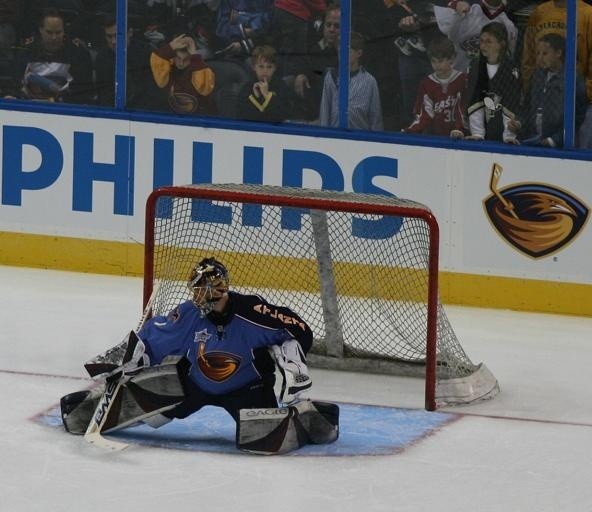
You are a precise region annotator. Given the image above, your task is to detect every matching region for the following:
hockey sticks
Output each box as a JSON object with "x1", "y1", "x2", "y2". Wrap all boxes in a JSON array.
[
  {"x1": 197, "y1": 342, "x2": 210, "y2": 366},
  {"x1": 82, "y1": 283, "x2": 162, "y2": 452},
  {"x1": 489, "y1": 162, "x2": 518, "y2": 219}
]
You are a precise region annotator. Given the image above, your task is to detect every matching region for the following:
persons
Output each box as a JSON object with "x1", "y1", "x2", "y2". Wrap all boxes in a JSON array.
[
  {"x1": 1, "y1": 1, "x2": 591, "y2": 150},
  {"x1": 61, "y1": 259, "x2": 339, "y2": 456}
]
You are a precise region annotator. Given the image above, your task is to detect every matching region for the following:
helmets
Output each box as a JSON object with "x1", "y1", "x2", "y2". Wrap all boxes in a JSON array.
[{"x1": 187, "y1": 258, "x2": 229, "y2": 316}]
